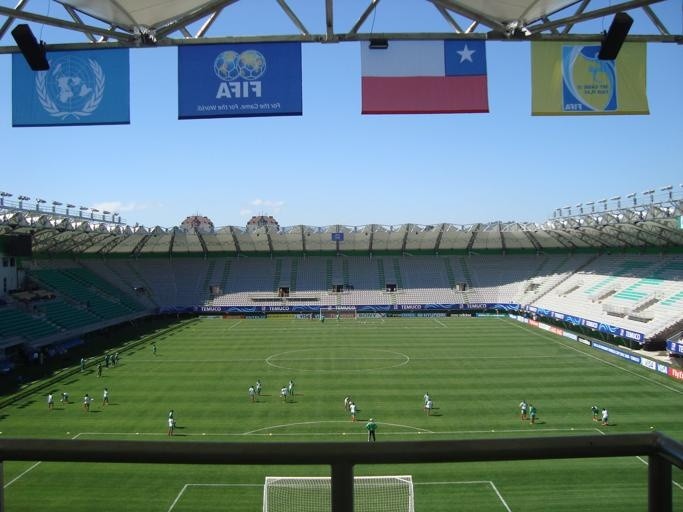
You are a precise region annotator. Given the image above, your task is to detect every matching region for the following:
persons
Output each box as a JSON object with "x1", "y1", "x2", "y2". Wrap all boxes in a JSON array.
[
  {"x1": 288, "y1": 379, "x2": 295, "y2": 396},
  {"x1": 60, "y1": 390, "x2": 70, "y2": 404},
  {"x1": 280, "y1": 385, "x2": 287, "y2": 400},
  {"x1": 591, "y1": 404, "x2": 599, "y2": 423},
  {"x1": 80, "y1": 357, "x2": 84, "y2": 372},
  {"x1": 527, "y1": 404, "x2": 536, "y2": 424},
  {"x1": 248, "y1": 385, "x2": 254, "y2": 403},
  {"x1": 601, "y1": 407, "x2": 609, "y2": 427},
  {"x1": 335, "y1": 307, "x2": 340, "y2": 322},
  {"x1": 151, "y1": 345, "x2": 160, "y2": 360},
  {"x1": 95, "y1": 363, "x2": 101, "y2": 378},
  {"x1": 109, "y1": 353, "x2": 114, "y2": 366},
  {"x1": 320, "y1": 313, "x2": 325, "y2": 325},
  {"x1": 168, "y1": 409, "x2": 175, "y2": 427},
  {"x1": 104, "y1": 354, "x2": 108, "y2": 366},
  {"x1": 255, "y1": 379, "x2": 261, "y2": 396},
  {"x1": 344, "y1": 395, "x2": 351, "y2": 416},
  {"x1": 101, "y1": 386, "x2": 109, "y2": 408},
  {"x1": 365, "y1": 417, "x2": 377, "y2": 443},
  {"x1": 518, "y1": 399, "x2": 527, "y2": 422},
  {"x1": 114, "y1": 352, "x2": 118, "y2": 365},
  {"x1": 167, "y1": 415, "x2": 173, "y2": 437},
  {"x1": 46, "y1": 391, "x2": 55, "y2": 410},
  {"x1": 423, "y1": 391, "x2": 429, "y2": 404},
  {"x1": 349, "y1": 402, "x2": 356, "y2": 422},
  {"x1": 82, "y1": 394, "x2": 90, "y2": 414},
  {"x1": 423, "y1": 398, "x2": 432, "y2": 416}
]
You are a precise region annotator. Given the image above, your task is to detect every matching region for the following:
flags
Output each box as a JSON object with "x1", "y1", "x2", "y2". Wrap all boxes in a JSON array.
[
  {"x1": 529, "y1": 35, "x2": 654, "y2": 117},
  {"x1": 9, "y1": 48, "x2": 132, "y2": 129},
  {"x1": 174, "y1": 44, "x2": 304, "y2": 121},
  {"x1": 357, "y1": 34, "x2": 493, "y2": 118}
]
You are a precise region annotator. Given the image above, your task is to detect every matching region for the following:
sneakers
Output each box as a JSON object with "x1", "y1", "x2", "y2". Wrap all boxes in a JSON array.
[
  {"x1": 521, "y1": 415, "x2": 535, "y2": 425},
  {"x1": 592, "y1": 416, "x2": 609, "y2": 426}
]
